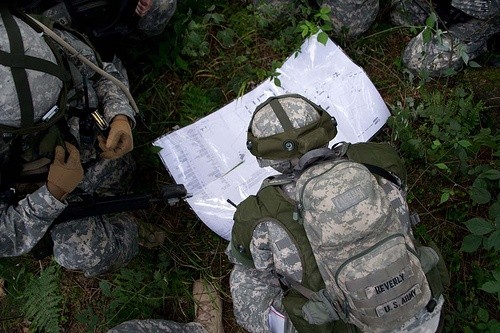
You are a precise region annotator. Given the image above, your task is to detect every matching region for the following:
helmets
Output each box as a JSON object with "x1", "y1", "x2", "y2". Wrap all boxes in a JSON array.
[
  {"x1": 247, "y1": 93, "x2": 338, "y2": 167},
  {"x1": 0, "y1": 15, "x2": 62, "y2": 128}
]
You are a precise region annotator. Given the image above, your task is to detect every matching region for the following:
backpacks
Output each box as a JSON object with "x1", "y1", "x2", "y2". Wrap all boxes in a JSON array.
[{"x1": 296, "y1": 160, "x2": 431, "y2": 332}]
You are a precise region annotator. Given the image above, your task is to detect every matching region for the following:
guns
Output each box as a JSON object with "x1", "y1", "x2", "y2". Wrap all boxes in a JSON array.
[{"x1": 0, "y1": 184, "x2": 186, "y2": 261}]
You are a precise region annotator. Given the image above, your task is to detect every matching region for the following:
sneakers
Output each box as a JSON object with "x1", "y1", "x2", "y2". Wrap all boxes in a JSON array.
[
  {"x1": 193, "y1": 277, "x2": 222, "y2": 333},
  {"x1": 138, "y1": 222, "x2": 166, "y2": 248}
]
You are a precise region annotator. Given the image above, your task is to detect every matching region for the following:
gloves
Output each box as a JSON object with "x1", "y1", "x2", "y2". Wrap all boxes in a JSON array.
[
  {"x1": 48, "y1": 142, "x2": 84, "y2": 200},
  {"x1": 97, "y1": 115, "x2": 133, "y2": 160}
]
